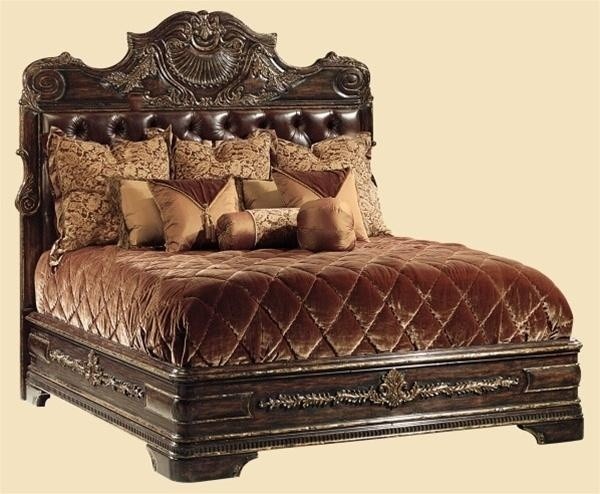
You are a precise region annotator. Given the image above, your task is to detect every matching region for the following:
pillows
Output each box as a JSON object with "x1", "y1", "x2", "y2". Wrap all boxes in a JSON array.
[
  {"x1": 295, "y1": 198, "x2": 357, "y2": 252},
  {"x1": 214, "y1": 207, "x2": 299, "y2": 247},
  {"x1": 169, "y1": 129, "x2": 275, "y2": 179},
  {"x1": 273, "y1": 167, "x2": 371, "y2": 243},
  {"x1": 48, "y1": 127, "x2": 174, "y2": 268},
  {"x1": 274, "y1": 132, "x2": 391, "y2": 234},
  {"x1": 239, "y1": 176, "x2": 283, "y2": 210},
  {"x1": 112, "y1": 177, "x2": 167, "y2": 249},
  {"x1": 145, "y1": 173, "x2": 241, "y2": 255}
]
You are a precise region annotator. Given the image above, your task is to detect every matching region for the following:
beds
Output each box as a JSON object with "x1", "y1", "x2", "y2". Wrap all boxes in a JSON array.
[{"x1": 14, "y1": 8, "x2": 585, "y2": 483}]
